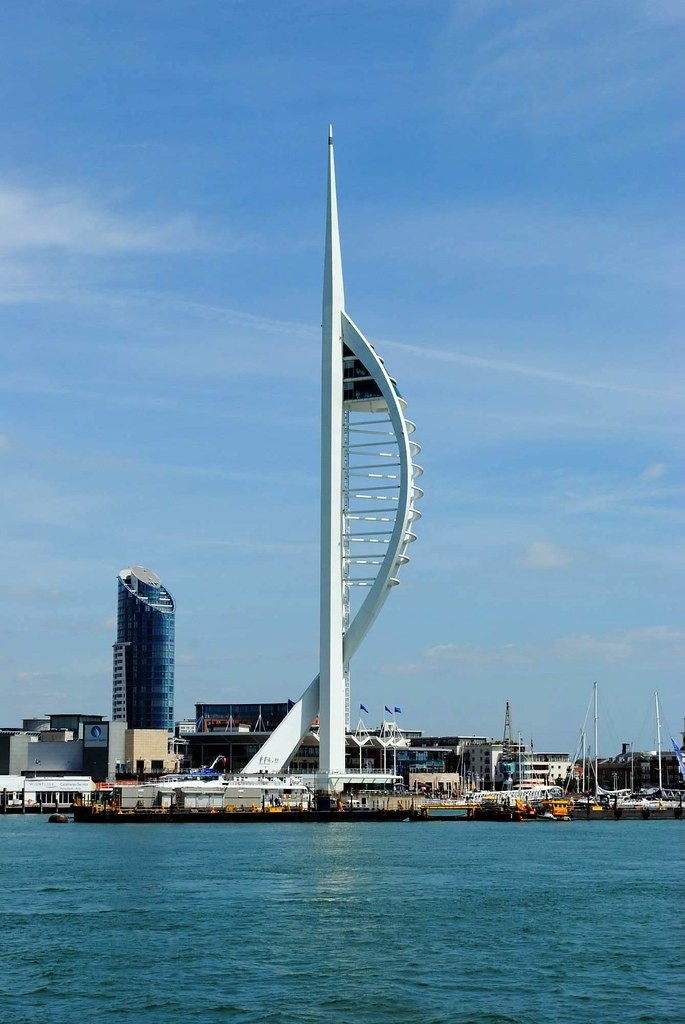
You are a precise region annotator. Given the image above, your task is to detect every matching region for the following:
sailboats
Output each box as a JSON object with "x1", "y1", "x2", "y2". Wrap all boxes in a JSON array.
[{"x1": 555, "y1": 682, "x2": 685, "y2": 811}]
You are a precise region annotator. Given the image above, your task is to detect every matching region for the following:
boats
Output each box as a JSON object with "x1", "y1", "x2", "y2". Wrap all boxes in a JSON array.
[
  {"x1": 70, "y1": 769, "x2": 419, "y2": 823},
  {"x1": 510, "y1": 797, "x2": 572, "y2": 821}
]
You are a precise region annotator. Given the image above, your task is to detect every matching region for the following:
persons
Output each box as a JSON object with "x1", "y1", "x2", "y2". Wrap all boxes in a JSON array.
[{"x1": 66, "y1": 776, "x2": 623, "y2": 818}]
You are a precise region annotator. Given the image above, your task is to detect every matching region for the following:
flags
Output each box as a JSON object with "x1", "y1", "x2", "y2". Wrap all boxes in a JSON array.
[
  {"x1": 384, "y1": 705, "x2": 393, "y2": 715},
  {"x1": 394, "y1": 706, "x2": 402, "y2": 713},
  {"x1": 530, "y1": 738, "x2": 535, "y2": 753},
  {"x1": 669, "y1": 737, "x2": 685, "y2": 783},
  {"x1": 358, "y1": 703, "x2": 369, "y2": 715}
]
